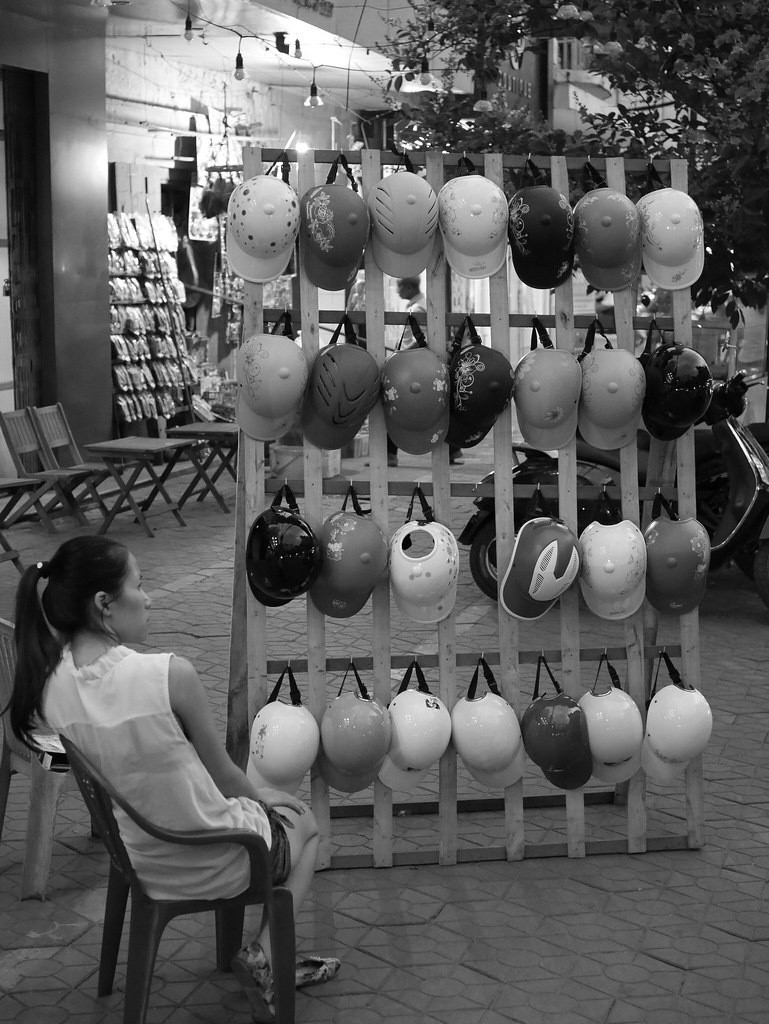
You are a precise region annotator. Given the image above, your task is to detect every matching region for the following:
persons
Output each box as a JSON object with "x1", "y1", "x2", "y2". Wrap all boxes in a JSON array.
[
  {"x1": 364, "y1": 275, "x2": 464, "y2": 468},
  {"x1": 0, "y1": 536, "x2": 319, "y2": 1024}
]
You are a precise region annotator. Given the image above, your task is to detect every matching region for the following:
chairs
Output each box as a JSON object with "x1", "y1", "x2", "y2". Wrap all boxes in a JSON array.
[
  {"x1": 31, "y1": 403, "x2": 136, "y2": 522},
  {"x1": 0, "y1": 476, "x2": 43, "y2": 575},
  {"x1": 60, "y1": 737, "x2": 296, "y2": 1024},
  {"x1": 0, "y1": 406, "x2": 96, "y2": 534},
  {"x1": 0, "y1": 618, "x2": 106, "y2": 903}
]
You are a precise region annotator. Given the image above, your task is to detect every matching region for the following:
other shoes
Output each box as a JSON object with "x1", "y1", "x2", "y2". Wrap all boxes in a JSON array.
[
  {"x1": 232, "y1": 941, "x2": 276, "y2": 1023},
  {"x1": 295, "y1": 957, "x2": 341, "y2": 986}
]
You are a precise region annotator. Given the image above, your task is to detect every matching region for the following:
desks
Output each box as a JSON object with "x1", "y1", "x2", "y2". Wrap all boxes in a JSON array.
[
  {"x1": 166, "y1": 423, "x2": 240, "y2": 515},
  {"x1": 81, "y1": 436, "x2": 197, "y2": 537}
]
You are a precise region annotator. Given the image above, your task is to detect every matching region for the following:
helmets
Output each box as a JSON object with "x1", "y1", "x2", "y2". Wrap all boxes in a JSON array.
[
  {"x1": 641, "y1": 341, "x2": 713, "y2": 441},
  {"x1": 514, "y1": 347, "x2": 582, "y2": 451},
  {"x1": 235, "y1": 333, "x2": 309, "y2": 441},
  {"x1": 300, "y1": 184, "x2": 370, "y2": 291},
  {"x1": 379, "y1": 347, "x2": 450, "y2": 455},
  {"x1": 573, "y1": 188, "x2": 642, "y2": 291},
  {"x1": 444, "y1": 344, "x2": 514, "y2": 448},
  {"x1": 577, "y1": 348, "x2": 646, "y2": 450},
  {"x1": 226, "y1": 175, "x2": 300, "y2": 284},
  {"x1": 299, "y1": 343, "x2": 380, "y2": 450},
  {"x1": 248, "y1": 683, "x2": 714, "y2": 792},
  {"x1": 500, "y1": 517, "x2": 583, "y2": 621},
  {"x1": 508, "y1": 185, "x2": 575, "y2": 289},
  {"x1": 389, "y1": 521, "x2": 459, "y2": 624},
  {"x1": 246, "y1": 506, "x2": 322, "y2": 607},
  {"x1": 636, "y1": 188, "x2": 704, "y2": 290},
  {"x1": 437, "y1": 175, "x2": 509, "y2": 278},
  {"x1": 644, "y1": 515, "x2": 711, "y2": 616},
  {"x1": 579, "y1": 520, "x2": 647, "y2": 621},
  {"x1": 367, "y1": 172, "x2": 438, "y2": 278},
  {"x1": 310, "y1": 511, "x2": 388, "y2": 618}
]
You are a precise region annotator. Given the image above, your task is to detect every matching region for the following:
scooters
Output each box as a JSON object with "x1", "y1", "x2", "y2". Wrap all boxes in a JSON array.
[{"x1": 455, "y1": 328, "x2": 769, "y2": 609}]
